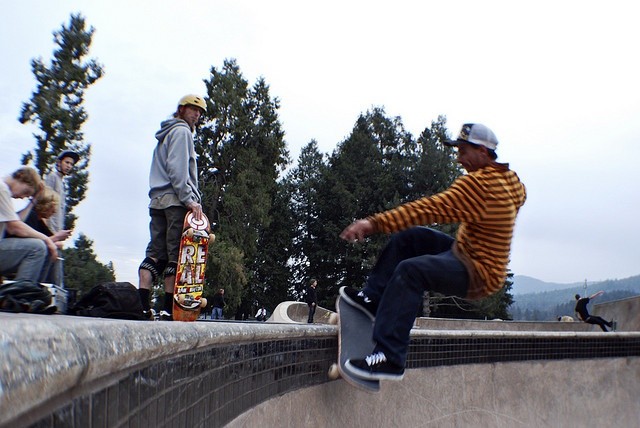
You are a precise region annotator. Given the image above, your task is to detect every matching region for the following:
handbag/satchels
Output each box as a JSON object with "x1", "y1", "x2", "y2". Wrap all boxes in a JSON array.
[
  {"x1": 0, "y1": 281, "x2": 51, "y2": 314},
  {"x1": 66, "y1": 282, "x2": 142, "y2": 320}
]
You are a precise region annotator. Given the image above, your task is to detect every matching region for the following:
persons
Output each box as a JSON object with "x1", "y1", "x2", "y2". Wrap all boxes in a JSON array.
[
  {"x1": 139, "y1": 95, "x2": 208, "y2": 315},
  {"x1": 307, "y1": 278, "x2": 318, "y2": 323},
  {"x1": 0, "y1": 167, "x2": 58, "y2": 281},
  {"x1": 44, "y1": 150, "x2": 79, "y2": 290},
  {"x1": 3, "y1": 186, "x2": 73, "y2": 287},
  {"x1": 339, "y1": 123, "x2": 526, "y2": 381},
  {"x1": 557, "y1": 316, "x2": 573, "y2": 321},
  {"x1": 574, "y1": 290, "x2": 613, "y2": 332},
  {"x1": 254, "y1": 305, "x2": 267, "y2": 321},
  {"x1": 211, "y1": 289, "x2": 226, "y2": 320}
]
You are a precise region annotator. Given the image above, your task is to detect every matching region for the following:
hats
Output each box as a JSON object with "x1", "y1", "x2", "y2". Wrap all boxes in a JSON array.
[{"x1": 442, "y1": 123, "x2": 499, "y2": 151}]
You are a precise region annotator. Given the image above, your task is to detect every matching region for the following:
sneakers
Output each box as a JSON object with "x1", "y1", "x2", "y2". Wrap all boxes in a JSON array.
[
  {"x1": 160, "y1": 311, "x2": 173, "y2": 321},
  {"x1": 339, "y1": 285, "x2": 380, "y2": 322},
  {"x1": 344, "y1": 351, "x2": 405, "y2": 382},
  {"x1": 143, "y1": 309, "x2": 156, "y2": 320}
]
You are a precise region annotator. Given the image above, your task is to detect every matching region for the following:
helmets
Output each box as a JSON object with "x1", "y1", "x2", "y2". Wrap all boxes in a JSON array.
[
  {"x1": 178, "y1": 93, "x2": 207, "y2": 113},
  {"x1": 57, "y1": 150, "x2": 79, "y2": 163}
]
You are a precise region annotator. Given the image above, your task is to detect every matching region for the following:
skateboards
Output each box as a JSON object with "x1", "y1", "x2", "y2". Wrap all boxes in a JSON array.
[
  {"x1": 171, "y1": 209, "x2": 217, "y2": 322},
  {"x1": 611, "y1": 316, "x2": 618, "y2": 331},
  {"x1": 327, "y1": 294, "x2": 381, "y2": 396}
]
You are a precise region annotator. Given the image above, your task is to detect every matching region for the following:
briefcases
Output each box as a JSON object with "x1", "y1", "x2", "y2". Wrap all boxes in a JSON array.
[{"x1": 2, "y1": 280, "x2": 68, "y2": 315}]
